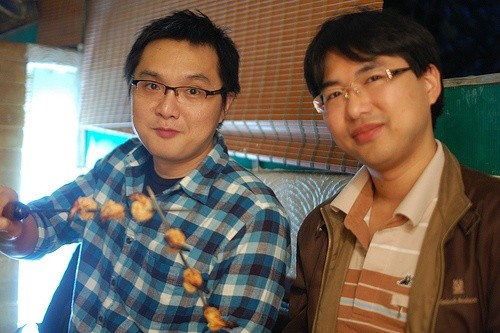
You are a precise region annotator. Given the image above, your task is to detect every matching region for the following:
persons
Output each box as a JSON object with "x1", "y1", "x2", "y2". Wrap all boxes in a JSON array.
[
  {"x1": 280, "y1": 9, "x2": 500, "y2": 333},
  {"x1": 0, "y1": 9, "x2": 291, "y2": 333}
]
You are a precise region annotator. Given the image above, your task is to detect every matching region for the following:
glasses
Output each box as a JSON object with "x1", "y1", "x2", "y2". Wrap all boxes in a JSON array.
[
  {"x1": 313, "y1": 66, "x2": 416, "y2": 115},
  {"x1": 127, "y1": 77, "x2": 224, "y2": 104}
]
